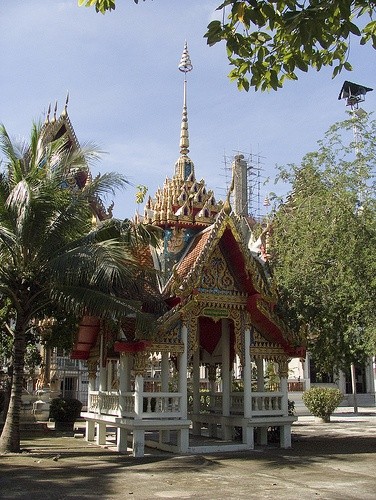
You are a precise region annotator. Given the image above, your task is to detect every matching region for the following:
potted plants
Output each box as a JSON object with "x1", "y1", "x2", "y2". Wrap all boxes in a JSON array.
[
  {"x1": 49, "y1": 397, "x2": 83, "y2": 436},
  {"x1": 301, "y1": 384, "x2": 345, "y2": 423}
]
